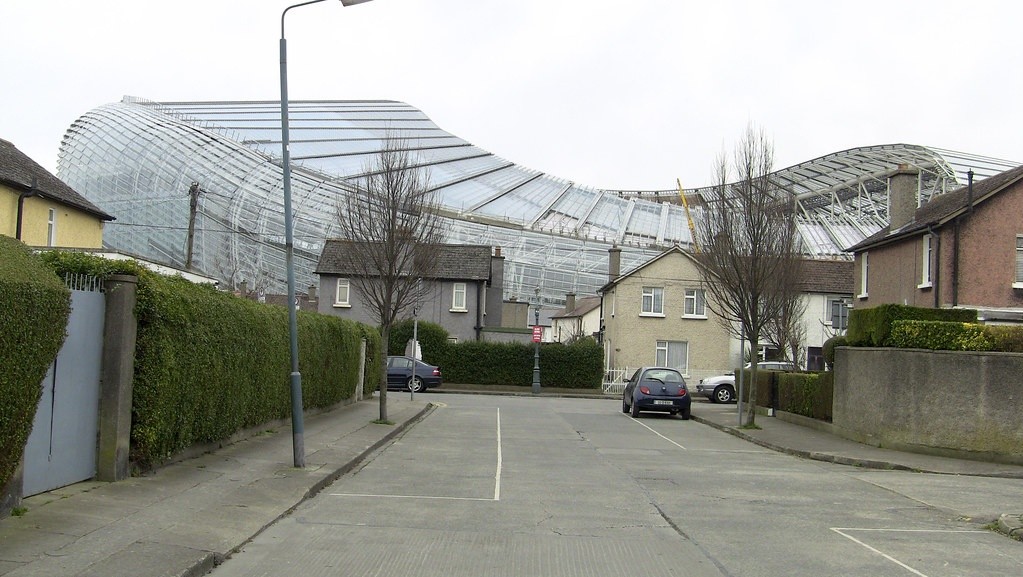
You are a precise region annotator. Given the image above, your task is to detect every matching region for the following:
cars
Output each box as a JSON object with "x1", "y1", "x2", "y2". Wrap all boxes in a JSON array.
[
  {"x1": 696, "y1": 358, "x2": 806, "y2": 404},
  {"x1": 376, "y1": 356, "x2": 442, "y2": 393},
  {"x1": 622, "y1": 366, "x2": 692, "y2": 420}
]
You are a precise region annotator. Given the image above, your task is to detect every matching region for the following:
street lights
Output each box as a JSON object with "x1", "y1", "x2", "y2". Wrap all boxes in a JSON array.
[{"x1": 280, "y1": 0, "x2": 373, "y2": 468}]
